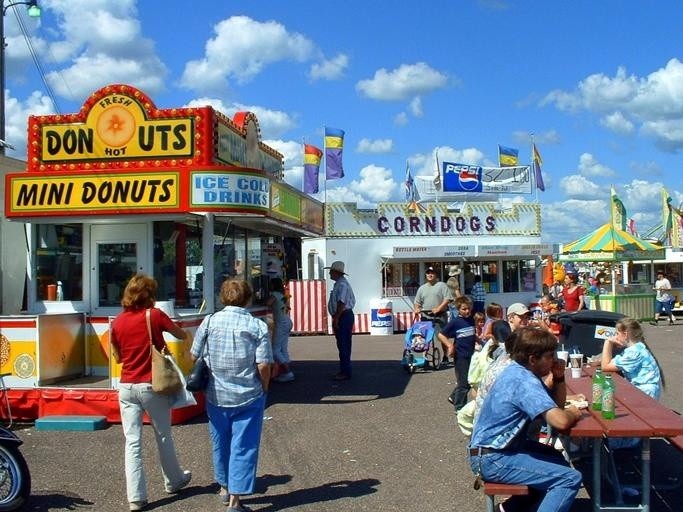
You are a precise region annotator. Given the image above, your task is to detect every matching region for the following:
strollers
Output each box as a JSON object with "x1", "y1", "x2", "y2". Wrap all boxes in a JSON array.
[{"x1": 401, "y1": 308, "x2": 441, "y2": 372}]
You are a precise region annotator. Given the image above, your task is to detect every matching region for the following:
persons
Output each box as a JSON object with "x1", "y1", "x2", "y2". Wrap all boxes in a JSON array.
[
  {"x1": 266, "y1": 270, "x2": 295, "y2": 382},
  {"x1": 597, "y1": 317, "x2": 661, "y2": 451},
  {"x1": 189, "y1": 278, "x2": 274, "y2": 511},
  {"x1": 557, "y1": 273, "x2": 584, "y2": 311},
  {"x1": 648, "y1": 271, "x2": 674, "y2": 326},
  {"x1": 108, "y1": 274, "x2": 198, "y2": 511},
  {"x1": 465, "y1": 328, "x2": 584, "y2": 511},
  {"x1": 412, "y1": 329, "x2": 424, "y2": 347},
  {"x1": 434, "y1": 296, "x2": 562, "y2": 433},
  {"x1": 321, "y1": 261, "x2": 355, "y2": 381},
  {"x1": 413, "y1": 266, "x2": 452, "y2": 363}
]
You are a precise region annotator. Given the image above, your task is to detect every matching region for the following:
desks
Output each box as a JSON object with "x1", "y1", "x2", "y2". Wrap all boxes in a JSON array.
[{"x1": 541, "y1": 363, "x2": 683, "y2": 512}]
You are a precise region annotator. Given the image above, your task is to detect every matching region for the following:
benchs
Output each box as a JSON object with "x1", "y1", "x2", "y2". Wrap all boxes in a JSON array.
[
  {"x1": 482, "y1": 481, "x2": 529, "y2": 511},
  {"x1": 664, "y1": 408, "x2": 683, "y2": 451}
]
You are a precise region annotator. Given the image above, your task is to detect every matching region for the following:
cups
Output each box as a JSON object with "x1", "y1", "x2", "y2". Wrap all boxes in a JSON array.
[
  {"x1": 107, "y1": 284, "x2": 121, "y2": 302},
  {"x1": 47, "y1": 284, "x2": 56, "y2": 300},
  {"x1": 556, "y1": 350, "x2": 568, "y2": 368},
  {"x1": 570, "y1": 354, "x2": 584, "y2": 378}
]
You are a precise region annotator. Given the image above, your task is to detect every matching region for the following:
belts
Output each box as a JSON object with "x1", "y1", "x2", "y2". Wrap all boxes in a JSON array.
[{"x1": 467, "y1": 442, "x2": 514, "y2": 456}]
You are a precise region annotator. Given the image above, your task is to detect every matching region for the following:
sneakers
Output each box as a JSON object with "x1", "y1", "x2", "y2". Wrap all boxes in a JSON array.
[
  {"x1": 649, "y1": 320, "x2": 658, "y2": 326},
  {"x1": 219, "y1": 491, "x2": 230, "y2": 506},
  {"x1": 165, "y1": 469, "x2": 193, "y2": 493},
  {"x1": 331, "y1": 369, "x2": 351, "y2": 381},
  {"x1": 447, "y1": 394, "x2": 463, "y2": 415},
  {"x1": 128, "y1": 500, "x2": 150, "y2": 510},
  {"x1": 498, "y1": 501, "x2": 513, "y2": 512},
  {"x1": 441, "y1": 354, "x2": 448, "y2": 365},
  {"x1": 272, "y1": 370, "x2": 294, "y2": 382},
  {"x1": 226, "y1": 506, "x2": 252, "y2": 512},
  {"x1": 670, "y1": 320, "x2": 674, "y2": 326}
]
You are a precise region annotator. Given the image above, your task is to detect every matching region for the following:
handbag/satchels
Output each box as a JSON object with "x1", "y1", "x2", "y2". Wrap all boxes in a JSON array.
[
  {"x1": 187, "y1": 347, "x2": 209, "y2": 394},
  {"x1": 151, "y1": 345, "x2": 183, "y2": 397},
  {"x1": 165, "y1": 351, "x2": 199, "y2": 409}
]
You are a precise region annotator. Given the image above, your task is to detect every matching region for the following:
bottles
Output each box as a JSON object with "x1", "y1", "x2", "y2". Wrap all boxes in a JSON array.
[
  {"x1": 592, "y1": 368, "x2": 604, "y2": 411},
  {"x1": 599, "y1": 375, "x2": 617, "y2": 422}
]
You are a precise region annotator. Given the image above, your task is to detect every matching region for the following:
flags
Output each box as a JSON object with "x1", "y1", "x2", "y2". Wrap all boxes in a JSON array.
[
  {"x1": 404, "y1": 159, "x2": 420, "y2": 203},
  {"x1": 662, "y1": 190, "x2": 672, "y2": 237},
  {"x1": 433, "y1": 151, "x2": 441, "y2": 192},
  {"x1": 611, "y1": 186, "x2": 626, "y2": 231},
  {"x1": 324, "y1": 127, "x2": 344, "y2": 180},
  {"x1": 497, "y1": 144, "x2": 518, "y2": 168},
  {"x1": 532, "y1": 143, "x2": 544, "y2": 192},
  {"x1": 302, "y1": 143, "x2": 324, "y2": 194},
  {"x1": 669, "y1": 210, "x2": 682, "y2": 247}
]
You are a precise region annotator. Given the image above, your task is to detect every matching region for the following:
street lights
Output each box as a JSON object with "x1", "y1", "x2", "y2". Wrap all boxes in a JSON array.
[{"x1": 0, "y1": 0, "x2": 41, "y2": 159}]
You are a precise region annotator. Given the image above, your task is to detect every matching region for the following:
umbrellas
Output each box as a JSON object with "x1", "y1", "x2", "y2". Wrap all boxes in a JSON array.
[{"x1": 560, "y1": 224, "x2": 672, "y2": 296}]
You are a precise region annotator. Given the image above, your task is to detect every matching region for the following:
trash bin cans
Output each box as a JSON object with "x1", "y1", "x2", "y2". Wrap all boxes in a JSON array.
[{"x1": 549, "y1": 309, "x2": 628, "y2": 367}]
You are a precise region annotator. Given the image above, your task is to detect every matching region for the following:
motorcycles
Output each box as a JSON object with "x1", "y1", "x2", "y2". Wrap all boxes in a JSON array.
[{"x1": 0, "y1": 373, "x2": 36, "y2": 512}]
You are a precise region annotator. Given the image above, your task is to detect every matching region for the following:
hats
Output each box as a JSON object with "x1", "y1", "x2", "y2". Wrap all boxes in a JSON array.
[
  {"x1": 448, "y1": 265, "x2": 462, "y2": 277},
  {"x1": 507, "y1": 302, "x2": 535, "y2": 317},
  {"x1": 425, "y1": 265, "x2": 437, "y2": 274},
  {"x1": 323, "y1": 261, "x2": 350, "y2": 276}
]
